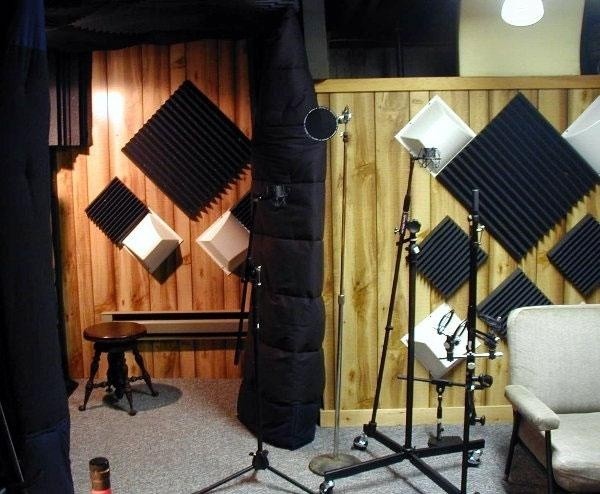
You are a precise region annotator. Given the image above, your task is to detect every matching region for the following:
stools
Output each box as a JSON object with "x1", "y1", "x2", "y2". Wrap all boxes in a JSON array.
[{"x1": 77, "y1": 322, "x2": 159, "y2": 416}]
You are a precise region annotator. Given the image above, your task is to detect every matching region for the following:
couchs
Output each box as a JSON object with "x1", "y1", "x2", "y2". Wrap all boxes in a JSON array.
[{"x1": 503, "y1": 304, "x2": 600, "y2": 494}]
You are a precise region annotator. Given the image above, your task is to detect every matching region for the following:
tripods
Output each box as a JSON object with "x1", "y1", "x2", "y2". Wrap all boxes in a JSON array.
[
  {"x1": 191, "y1": 184, "x2": 314, "y2": 494},
  {"x1": 325, "y1": 156, "x2": 487, "y2": 493}
]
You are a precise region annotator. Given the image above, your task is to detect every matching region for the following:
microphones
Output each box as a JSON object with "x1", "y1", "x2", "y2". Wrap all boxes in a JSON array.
[
  {"x1": 302, "y1": 106, "x2": 351, "y2": 143},
  {"x1": 86, "y1": 453, "x2": 113, "y2": 492}
]
